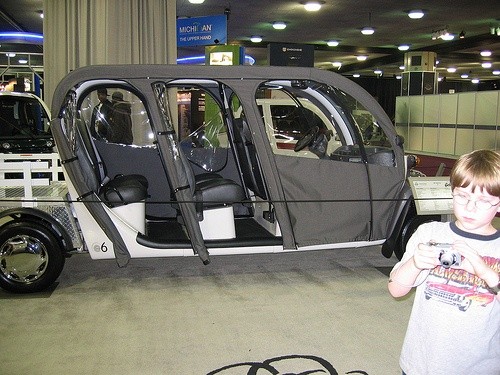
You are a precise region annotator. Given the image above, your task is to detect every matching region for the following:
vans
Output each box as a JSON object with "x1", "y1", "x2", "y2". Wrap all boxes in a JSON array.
[{"x1": 217, "y1": 98, "x2": 364, "y2": 161}]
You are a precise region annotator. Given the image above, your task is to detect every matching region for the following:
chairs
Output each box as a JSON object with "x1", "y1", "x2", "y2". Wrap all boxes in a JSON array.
[
  {"x1": 243, "y1": 120, "x2": 268, "y2": 200},
  {"x1": 230, "y1": 118, "x2": 257, "y2": 195}
]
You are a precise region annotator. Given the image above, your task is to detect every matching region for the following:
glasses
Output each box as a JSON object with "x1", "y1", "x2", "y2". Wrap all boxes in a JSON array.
[{"x1": 451, "y1": 193, "x2": 499, "y2": 210}]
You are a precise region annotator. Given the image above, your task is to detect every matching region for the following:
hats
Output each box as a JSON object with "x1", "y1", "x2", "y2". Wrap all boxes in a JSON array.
[
  {"x1": 97, "y1": 88, "x2": 111, "y2": 96},
  {"x1": 112, "y1": 92, "x2": 124, "y2": 101}
]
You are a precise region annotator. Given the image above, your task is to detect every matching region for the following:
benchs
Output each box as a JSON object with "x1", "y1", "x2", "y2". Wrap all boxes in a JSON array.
[
  {"x1": 153, "y1": 129, "x2": 246, "y2": 207},
  {"x1": 78, "y1": 121, "x2": 149, "y2": 204}
]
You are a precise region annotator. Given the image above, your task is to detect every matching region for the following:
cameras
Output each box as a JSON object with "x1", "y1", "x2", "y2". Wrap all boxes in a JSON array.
[{"x1": 427, "y1": 242, "x2": 462, "y2": 268}]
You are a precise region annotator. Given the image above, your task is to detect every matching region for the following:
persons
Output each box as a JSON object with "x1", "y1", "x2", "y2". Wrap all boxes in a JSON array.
[
  {"x1": 388, "y1": 149, "x2": 500, "y2": 375},
  {"x1": 90, "y1": 87, "x2": 133, "y2": 144}
]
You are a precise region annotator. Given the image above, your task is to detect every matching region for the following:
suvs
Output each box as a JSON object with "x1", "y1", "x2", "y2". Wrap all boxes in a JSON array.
[{"x1": 0, "y1": 91, "x2": 63, "y2": 184}]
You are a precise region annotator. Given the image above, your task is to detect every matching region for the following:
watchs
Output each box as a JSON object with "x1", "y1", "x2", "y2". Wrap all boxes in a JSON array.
[{"x1": 485, "y1": 283, "x2": 500, "y2": 293}]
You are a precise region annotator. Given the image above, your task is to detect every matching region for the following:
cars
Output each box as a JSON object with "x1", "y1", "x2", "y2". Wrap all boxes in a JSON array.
[{"x1": 0, "y1": 64, "x2": 441, "y2": 293}]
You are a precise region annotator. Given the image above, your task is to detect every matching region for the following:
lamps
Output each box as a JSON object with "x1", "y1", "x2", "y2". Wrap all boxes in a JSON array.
[
  {"x1": 408, "y1": 10, "x2": 424, "y2": 18},
  {"x1": 326, "y1": 27, "x2": 382, "y2": 77},
  {"x1": 304, "y1": 0, "x2": 320, "y2": 11},
  {"x1": 250, "y1": 35, "x2": 262, "y2": 42},
  {"x1": 396, "y1": 30, "x2": 500, "y2": 83},
  {"x1": 273, "y1": 22, "x2": 286, "y2": 30}
]
